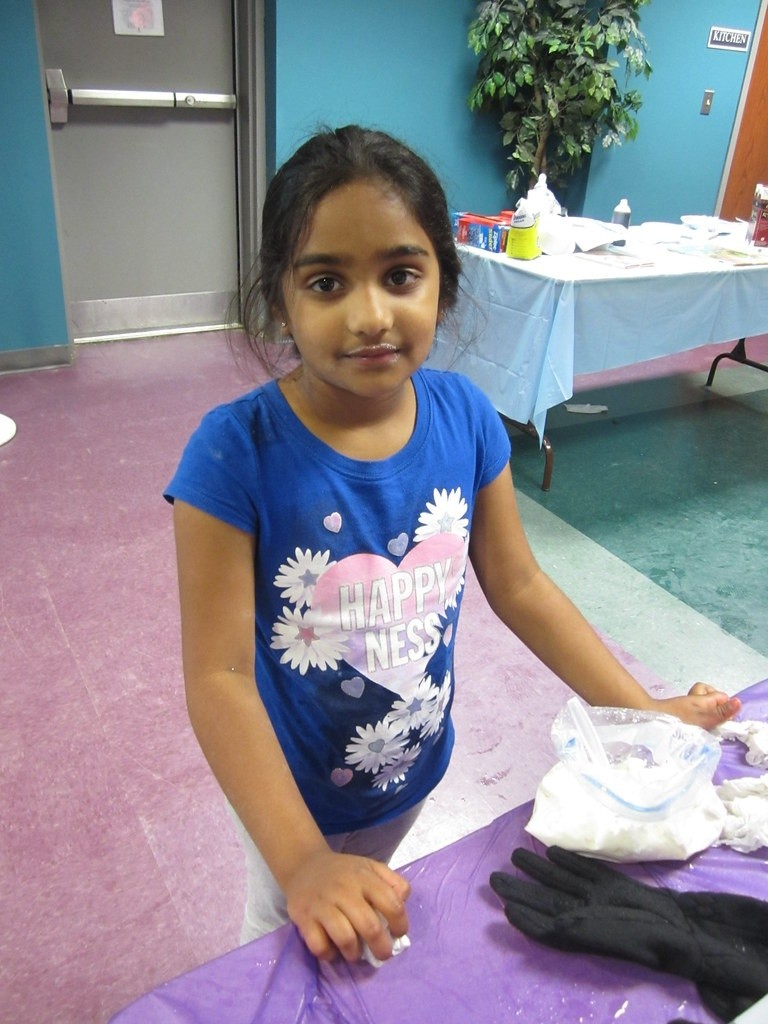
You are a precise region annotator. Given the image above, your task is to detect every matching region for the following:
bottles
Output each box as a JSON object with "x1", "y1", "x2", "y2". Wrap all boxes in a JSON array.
[{"x1": 607, "y1": 198, "x2": 632, "y2": 246}]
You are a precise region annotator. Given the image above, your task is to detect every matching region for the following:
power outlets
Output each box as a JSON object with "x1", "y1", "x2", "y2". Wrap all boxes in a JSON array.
[{"x1": 699, "y1": 89, "x2": 713, "y2": 115}]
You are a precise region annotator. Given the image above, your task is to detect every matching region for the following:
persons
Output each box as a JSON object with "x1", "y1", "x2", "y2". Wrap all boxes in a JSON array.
[{"x1": 161, "y1": 123, "x2": 742, "y2": 964}]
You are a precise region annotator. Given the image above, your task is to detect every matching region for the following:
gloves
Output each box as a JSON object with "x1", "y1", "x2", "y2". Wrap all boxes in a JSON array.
[{"x1": 491, "y1": 845, "x2": 768, "y2": 1024}]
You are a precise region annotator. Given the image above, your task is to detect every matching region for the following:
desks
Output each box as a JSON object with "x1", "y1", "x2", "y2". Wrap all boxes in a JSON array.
[
  {"x1": 95, "y1": 675, "x2": 768, "y2": 1024},
  {"x1": 420, "y1": 214, "x2": 768, "y2": 491}
]
